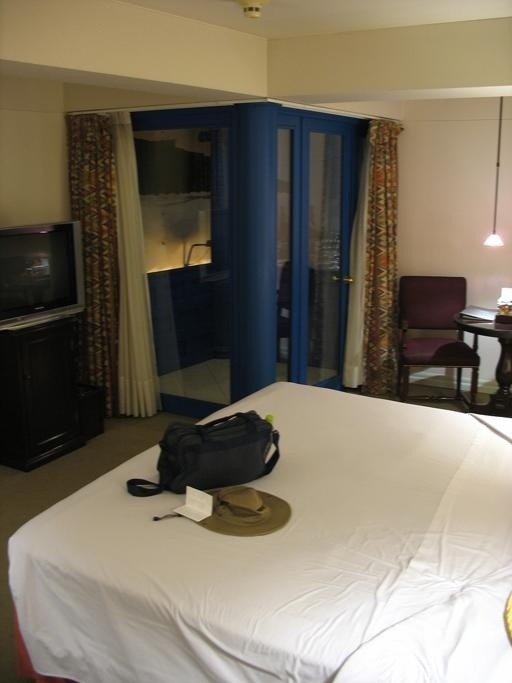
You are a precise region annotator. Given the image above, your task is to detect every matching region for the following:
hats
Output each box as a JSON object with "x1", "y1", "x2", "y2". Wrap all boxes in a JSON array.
[{"x1": 153, "y1": 486, "x2": 291, "y2": 537}]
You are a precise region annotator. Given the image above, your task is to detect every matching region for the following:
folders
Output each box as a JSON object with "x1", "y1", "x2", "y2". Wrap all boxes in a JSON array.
[{"x1": 459, "y1": 305, "x2": 499, "y2": 323}]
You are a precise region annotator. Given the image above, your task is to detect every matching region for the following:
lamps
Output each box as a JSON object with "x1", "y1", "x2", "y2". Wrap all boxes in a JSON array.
[{"x1": 484, "y1": 97, "x2": 506, "y2": 248}]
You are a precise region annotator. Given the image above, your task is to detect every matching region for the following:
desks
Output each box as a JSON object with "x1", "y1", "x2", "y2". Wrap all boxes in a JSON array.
[{"x1": 453, "y1": 308, "x2": 512, "y2": 418}]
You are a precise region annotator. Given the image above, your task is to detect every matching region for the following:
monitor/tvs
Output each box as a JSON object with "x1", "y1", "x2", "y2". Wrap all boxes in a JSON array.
[{"x1": 1, "y1": 219, "x2": 85, "y2": 330}]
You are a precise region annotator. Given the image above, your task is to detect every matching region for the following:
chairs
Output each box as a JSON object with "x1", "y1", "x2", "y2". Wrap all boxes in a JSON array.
[{"x1": 396, "y1": 275, "x2": 480, "y2": 412}]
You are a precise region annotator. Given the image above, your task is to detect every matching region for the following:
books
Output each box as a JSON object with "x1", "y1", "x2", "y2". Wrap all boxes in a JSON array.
[{"x1": 458, "y1": 304, "x2": 495, "y2": 323}]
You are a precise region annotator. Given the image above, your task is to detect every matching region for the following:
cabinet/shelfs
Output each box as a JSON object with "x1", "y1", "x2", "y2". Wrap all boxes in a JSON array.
[{"x1": 0, "y1": 318, "x2": 87, "y2": 472}]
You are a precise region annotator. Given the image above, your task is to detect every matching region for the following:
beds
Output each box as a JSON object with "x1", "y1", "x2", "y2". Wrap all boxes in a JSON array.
[{"x1": 7, "y1": 379, "x2": 512, "y2": 683}]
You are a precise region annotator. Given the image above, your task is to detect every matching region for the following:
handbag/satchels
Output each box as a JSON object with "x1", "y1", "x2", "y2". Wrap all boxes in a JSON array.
[{"x1": 127, "y1": 410, "x2": 280, "y2": 497}]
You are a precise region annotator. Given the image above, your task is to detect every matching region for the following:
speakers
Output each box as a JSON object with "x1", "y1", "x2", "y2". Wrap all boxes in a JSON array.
[{"x1": 78, "y1": 384, "x2": 106, "y2": 439}]
